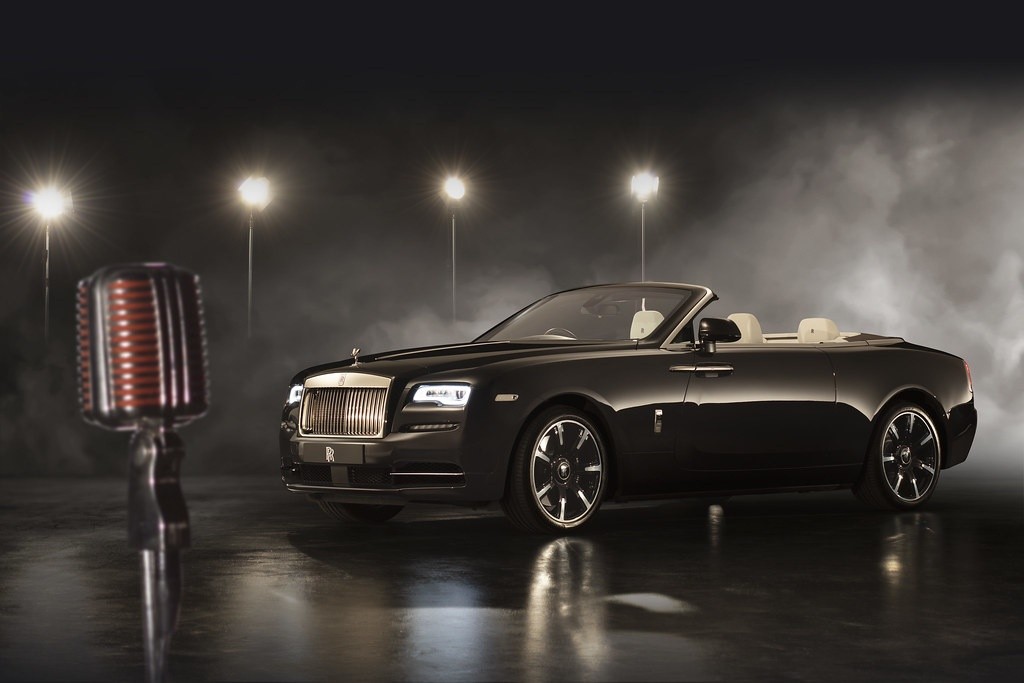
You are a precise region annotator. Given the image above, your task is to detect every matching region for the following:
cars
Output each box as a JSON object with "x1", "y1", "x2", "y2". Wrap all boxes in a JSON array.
[{"x1": 278, "y1": 279, "x2": 977, "y2": 533}]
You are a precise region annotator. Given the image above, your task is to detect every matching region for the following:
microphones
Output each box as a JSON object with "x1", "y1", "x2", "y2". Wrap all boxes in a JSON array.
[{"x1": 78, "y1": 260, "x2": 212, "y2": 644}]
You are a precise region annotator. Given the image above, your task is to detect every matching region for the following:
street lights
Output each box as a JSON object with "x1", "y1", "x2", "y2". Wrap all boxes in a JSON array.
[
  {"x1": 442, "y1": 172, "x2": 467, "y2": 342},
  {"x1": 238, "y1": 174, "x2": 272, "y2": 339},
  {"x1": 631, "y1": 174, "x2": 659, "y2": 281},
  {"x1": 31, "y1": 172, "x2": 74, "y2": 341}
]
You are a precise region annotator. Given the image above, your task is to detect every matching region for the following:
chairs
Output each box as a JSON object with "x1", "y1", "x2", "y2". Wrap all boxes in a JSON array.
[
  {"x1": 797, "y1": 318, "x2": 848, "y2": 343},
  {"x1": 727, "y1": 312, "x2": 768, "y2": 343},
  {"x1": 630, "y1": 311, "x2": 665, "y2": 339}
]
